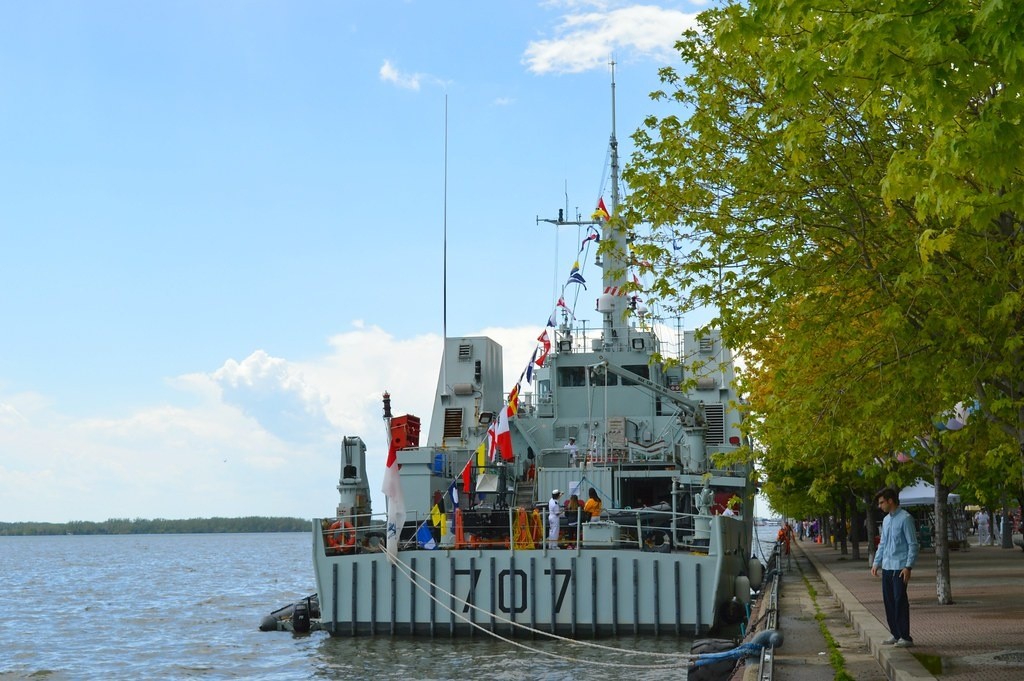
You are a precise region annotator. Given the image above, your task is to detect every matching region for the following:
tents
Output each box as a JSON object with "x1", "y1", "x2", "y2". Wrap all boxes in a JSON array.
[{"x1": 877, "y1": 476, "x2": 960, "y2": 508}]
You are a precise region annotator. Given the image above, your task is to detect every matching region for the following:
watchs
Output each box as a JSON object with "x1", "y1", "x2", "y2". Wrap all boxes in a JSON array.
[{"x1": 904, "y1": 566, "x2": 913, "y2": 571}]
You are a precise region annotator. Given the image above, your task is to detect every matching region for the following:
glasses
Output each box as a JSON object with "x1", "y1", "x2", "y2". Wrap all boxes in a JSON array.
[{"x1": 877, "y1": 500, "x2": 887, "y2": 507}]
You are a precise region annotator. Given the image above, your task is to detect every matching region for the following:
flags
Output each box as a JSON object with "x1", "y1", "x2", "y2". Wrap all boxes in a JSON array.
[
  {"x1": 475, "y1": 383, "x2": 521, "y2": 474},
  {"x1": 526, "y1": 295, "x2": 576, "y2": 385},
  {"x1": 416, "y1": 460, "x2": 472, "y2": 550},
  {"x1": 564, "y1": 196, "x2": 611, "y2": 290},
  {"x1": 628, "y1": 238, "x2": 653, "y2": 283},
  {"x1": 604, "y1": 285, "x2": 627, "y2": 296}
]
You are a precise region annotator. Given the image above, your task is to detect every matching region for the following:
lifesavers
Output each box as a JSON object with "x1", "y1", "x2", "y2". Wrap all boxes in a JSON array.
[
  {"x1": 328, "y1": 521, "x2": 356, "y2": 551},
  {"x1": 362, "y1": 532, "x2": 387, "y2": 553}
]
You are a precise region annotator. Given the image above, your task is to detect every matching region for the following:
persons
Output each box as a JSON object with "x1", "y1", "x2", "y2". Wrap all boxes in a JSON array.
[
  {"x1": 871, "y1": 487, "x2": 920, "y2": 647},
  {"x1": 970, "y1": 508, "x2": 1015, "y2": 547},
  {"x1": 583, "y1": 487, "x2": 602, "y2": 522},
  {"x1": 468, "y1": 474, "x2": 486, "y2": 512},
  {"x1": 563, "y1": 437, "x2": 576, "y2": 468},
  {"x1": 778, "y1": 521, "x2": 793, "y2": 555},
  {"x1": 564, "y1": 495, "x2": 582, "y2": 540},
  {"x1": 795, "y1": 516, "x2": 818, "y2": 541},
  {"x1": 548, "y1": 488, "x2": 564, "y2": 549}
]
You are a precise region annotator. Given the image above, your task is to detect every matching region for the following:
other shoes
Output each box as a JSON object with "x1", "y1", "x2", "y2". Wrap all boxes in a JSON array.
[
  {"x1": 549, "y1": 546, "x2": 560, "y2": 549},
  {"x1": 799, "y1": 538, "x2": 803, "y2": 541},
  {"x1": 567, "y1": 546, "x2": 572, "y2": 549},
  {"x1": 894, "y1": 638, "x2": 913, "y2": 647},
  {"x1": 881, "y1": 635, "x2": 898, "y2": 645}
]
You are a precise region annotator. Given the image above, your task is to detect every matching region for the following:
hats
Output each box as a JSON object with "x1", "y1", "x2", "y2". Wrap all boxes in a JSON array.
[
  {"x1": 552, "y1": 490, "x2": 558, "y2": 494},
  {"x1": 569, "y1": 437, "x2": 576, "y2": 441}
]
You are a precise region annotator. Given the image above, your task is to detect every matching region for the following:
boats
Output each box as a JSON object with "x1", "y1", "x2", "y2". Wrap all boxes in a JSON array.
[
  {"x1": 256, "y1": 590, "x2": 321, "y2": 633},
  {"x1": 310, "y1": 48, "x2": 760, "y2": 638}
]
